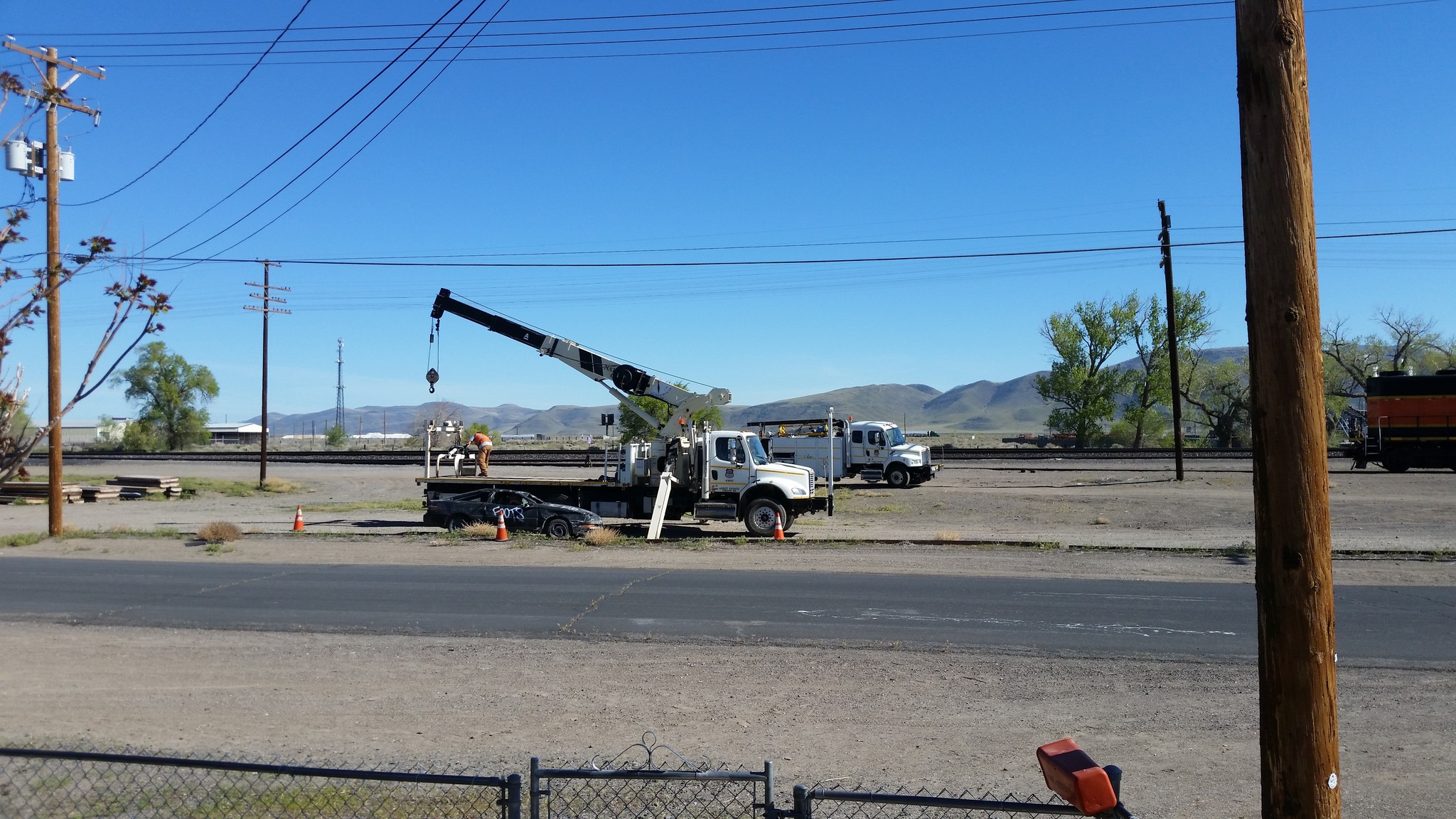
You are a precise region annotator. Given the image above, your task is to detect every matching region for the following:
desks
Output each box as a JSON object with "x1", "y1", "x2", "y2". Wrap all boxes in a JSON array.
[
  {"x1": 114, "y1": 474, "x2": 180, "y2": 488},
  {"x1": 0, "y1": 482, "x2": 85, "y2": 504}
]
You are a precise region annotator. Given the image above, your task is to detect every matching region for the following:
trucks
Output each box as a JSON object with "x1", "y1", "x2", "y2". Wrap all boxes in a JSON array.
[{"x1": 1338, "y1": 367, "x2": 1456, "y2": 474}]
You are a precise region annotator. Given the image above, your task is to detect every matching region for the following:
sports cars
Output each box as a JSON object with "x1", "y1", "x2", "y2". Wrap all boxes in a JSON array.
[{"x1": 421, "y1": 488, "x2": 603, "y2": 542}]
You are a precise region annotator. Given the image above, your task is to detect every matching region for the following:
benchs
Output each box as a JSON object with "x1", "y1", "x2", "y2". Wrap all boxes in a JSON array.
[
  {"x1": 0, "y1": 496, "x2": 46, "y2": 505},
  {"x1": 79, "y1": 484, "x2": 182, "y2": 502}
]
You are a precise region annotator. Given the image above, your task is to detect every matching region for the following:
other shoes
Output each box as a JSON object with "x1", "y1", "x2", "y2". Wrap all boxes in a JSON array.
[{"x1": 479, "y1": 473, "x2": 488, "y2": 477}]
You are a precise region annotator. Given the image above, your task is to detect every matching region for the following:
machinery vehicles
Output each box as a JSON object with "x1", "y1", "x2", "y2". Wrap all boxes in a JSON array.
[
  {"x1": 410, "y1": 288, "x2": 835, "y2": 537},
  {"x1": 745, "y1": 406, "x2": 946, "y2": 488}
]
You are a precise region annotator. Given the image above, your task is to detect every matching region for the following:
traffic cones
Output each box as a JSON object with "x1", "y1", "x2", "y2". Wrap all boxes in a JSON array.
[
  {"x1": 770, "y1": 512, "x2": 786, "y2": 540},
  {"x1": 289, "y1": 504, "x2": 309, "y2": 532},
  {"x1": 492, "y1": 509, "x2": 517, "y2": 542}
]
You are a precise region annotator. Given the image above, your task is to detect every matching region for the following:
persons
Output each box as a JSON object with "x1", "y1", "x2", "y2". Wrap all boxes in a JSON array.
[{"x1": 465, "y1": 431, "x2": 493, "y2": 477}]
